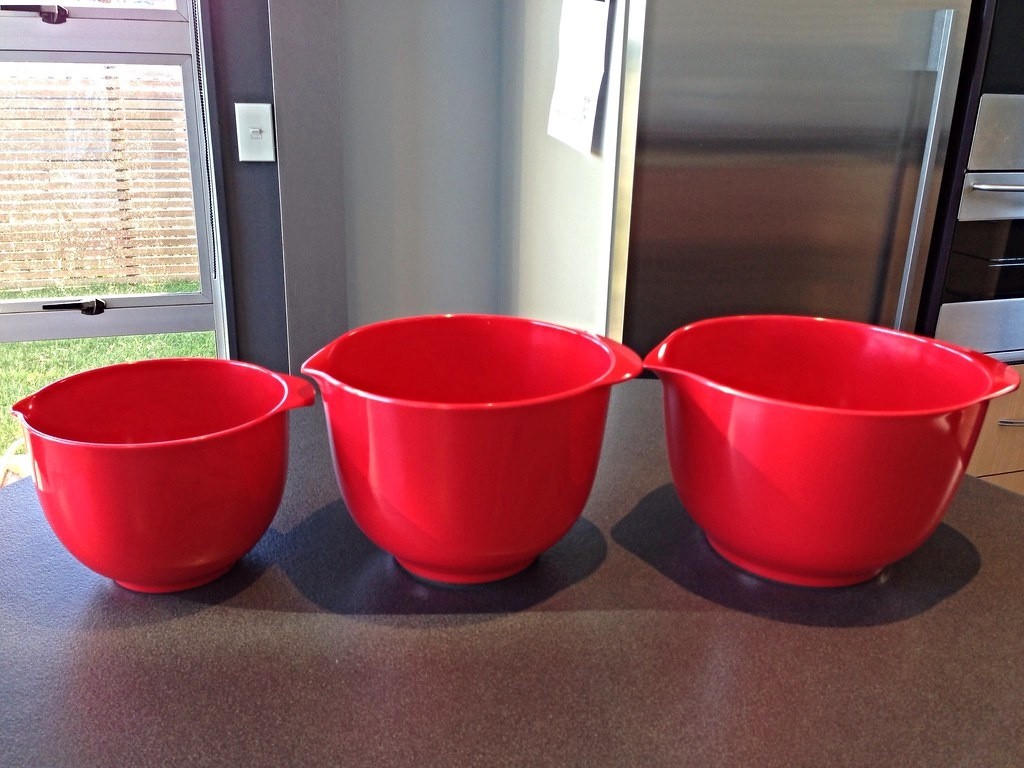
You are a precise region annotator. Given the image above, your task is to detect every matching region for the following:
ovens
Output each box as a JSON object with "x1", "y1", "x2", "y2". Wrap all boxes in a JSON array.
[{"x1": 913, "y1": 92, "x2": 1024, "y2": 363}]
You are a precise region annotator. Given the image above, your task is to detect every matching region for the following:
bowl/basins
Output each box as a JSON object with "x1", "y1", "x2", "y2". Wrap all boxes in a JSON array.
[
  {"x1": 640, "y1": 313, "x2": 1022, "y2": 589},
  {"x1": 300, "y1": 311, "x2": 641, "y2": 590},
  {"x1": 10, "y1": 353, "x2": 318, "y2": 595}
]
